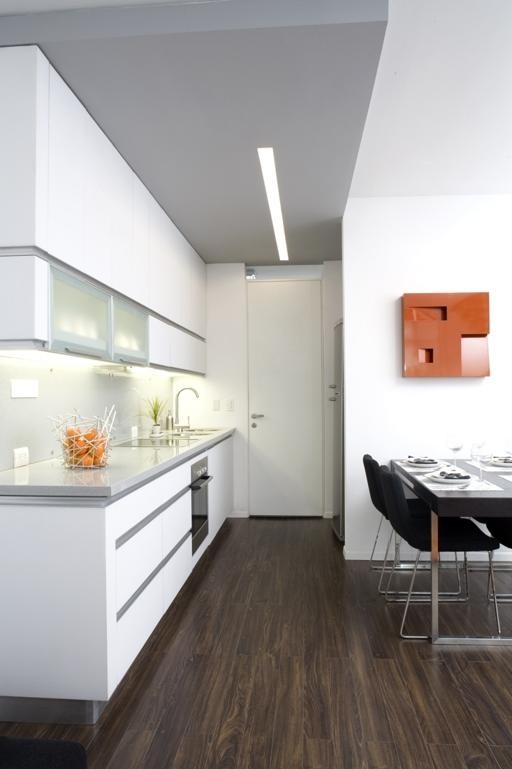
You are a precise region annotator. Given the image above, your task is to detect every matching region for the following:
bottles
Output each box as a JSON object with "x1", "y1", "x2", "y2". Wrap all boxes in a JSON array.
[{"x1": 165, "y1": 409, "x2": 173, "y2": 431}]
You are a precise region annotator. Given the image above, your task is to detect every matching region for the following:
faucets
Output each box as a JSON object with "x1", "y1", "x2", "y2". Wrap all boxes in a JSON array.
[{"x1": 174, "y1": 387, "x2": 200, "y2": 423}]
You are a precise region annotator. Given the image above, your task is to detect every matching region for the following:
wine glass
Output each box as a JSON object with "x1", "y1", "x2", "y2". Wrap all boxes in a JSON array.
[
  {"x1": 447, "y1": 436, "x2": 463, "y2": 469},
  {"x1": 470, "y1": 440, "x2": 493, "y2": 483}
]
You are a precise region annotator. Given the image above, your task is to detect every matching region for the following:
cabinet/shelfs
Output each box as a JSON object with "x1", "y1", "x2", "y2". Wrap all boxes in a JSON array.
[
  {"x1": 106, "y1": 434, "x2": 231, "y2": 709},
  {"x1": 31, "y1": 40, "x2": 213, "y2": 375}
]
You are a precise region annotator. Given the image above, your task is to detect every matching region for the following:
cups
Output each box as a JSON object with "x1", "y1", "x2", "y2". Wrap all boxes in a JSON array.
[{"x1": 151, "y1": 424, "x2": 161, "y2": 434}]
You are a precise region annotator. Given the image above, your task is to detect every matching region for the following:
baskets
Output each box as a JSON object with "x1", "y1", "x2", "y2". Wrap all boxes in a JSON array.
[{"x1": 49, "y1": 402, "x2": 118, "y2": 470}]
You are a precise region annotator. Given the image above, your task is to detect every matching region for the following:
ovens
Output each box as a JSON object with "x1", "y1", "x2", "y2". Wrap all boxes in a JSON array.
[{"x1": 190, "y1": 456, "x2": 213, "y2": 555}]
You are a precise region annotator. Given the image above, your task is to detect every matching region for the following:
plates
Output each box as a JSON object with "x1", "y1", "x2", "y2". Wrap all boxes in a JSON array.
[
  {"x1": 403, "y1": 459, "x2": 441, "y2": 469},
  {"x1": 490, "y1": 460, "x2": 511, "y2": 467},
  {"x1": 425, "y1": 473, "x2": 473, "y2": 484}
]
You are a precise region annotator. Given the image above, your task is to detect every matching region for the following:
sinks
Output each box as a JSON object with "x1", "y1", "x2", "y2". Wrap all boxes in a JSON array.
[{"x1": 171, "y1": 428, "x2": 220, "y2": 435}]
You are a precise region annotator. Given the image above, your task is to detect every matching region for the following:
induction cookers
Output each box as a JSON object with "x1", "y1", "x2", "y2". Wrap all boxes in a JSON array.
[{"x1": 113, "y1": 439, "x2": 200, "y2": 447}]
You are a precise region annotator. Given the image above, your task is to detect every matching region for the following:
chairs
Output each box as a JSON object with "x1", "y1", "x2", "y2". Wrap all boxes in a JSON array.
[{"x1": 363, "y1": 453, "x2": 503, "y2": 643}]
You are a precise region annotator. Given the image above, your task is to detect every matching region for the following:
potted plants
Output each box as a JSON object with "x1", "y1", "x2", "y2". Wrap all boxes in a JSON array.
[{"x1": 145, "y1": 394, "x2": 167, "y2": 434}]
